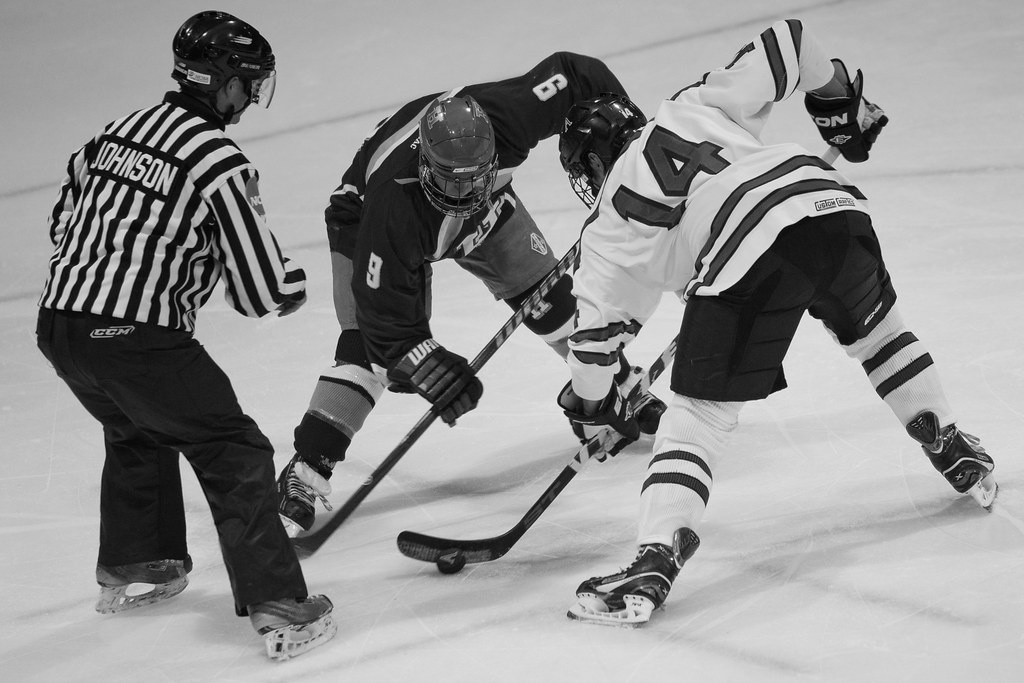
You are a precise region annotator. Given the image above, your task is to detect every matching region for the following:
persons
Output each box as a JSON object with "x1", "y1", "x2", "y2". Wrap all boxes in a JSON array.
[
  {"x1": 36, "y1": 10, "x2": 339, "y2": 661},
  {"x1": 558, "y1": 17, "x2": 998, "y2": 623},
  {"x1": 272, "y1": 51, "x2": 667, "y2": 538}
]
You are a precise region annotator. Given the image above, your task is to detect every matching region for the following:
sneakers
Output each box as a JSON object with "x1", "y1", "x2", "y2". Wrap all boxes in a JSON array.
[
  {"x1": 248, "y1": 593, "x2": 338, "y2": 662},
  {"x1": 631, "y1": 392, "x2": 668, "y2": 434},
  {"x1": 908, "y1": 409, "x2": 998, "y2": 513},
  {"x1": 95, "y1": 555, "x2": 192, "y2": 615},
  {"x1": 566, "y1": 527, "x2": 699, "y2": 630},
  {"x1": 277, "y1": 451, "x2": 336, "y2": 538}
]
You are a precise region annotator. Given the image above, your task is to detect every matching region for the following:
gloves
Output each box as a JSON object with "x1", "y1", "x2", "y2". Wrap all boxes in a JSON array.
[
  {"x1": 807, "y1": 59, "x2": 888, "y2": 163},
  {"x1": 558, "y1": 381, "x2": 639, "y2": 463},
  {"x1": 387, "y1": 337, "x2": 483, "y2": 428}
]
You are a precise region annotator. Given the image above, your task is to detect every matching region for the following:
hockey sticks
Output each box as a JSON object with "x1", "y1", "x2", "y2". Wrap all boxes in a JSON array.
[
  {"x1": 287, "y1": 239, "x2": 580, "y2": 556},
  {"x1": 395, "y1": 146, "x2": 838, "y2": 564}
]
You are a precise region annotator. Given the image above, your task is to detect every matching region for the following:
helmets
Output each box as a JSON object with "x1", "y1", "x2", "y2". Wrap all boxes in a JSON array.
[
  {"x1": 560, "y1": 92, "x2": 648, "y2": 210},
  {"x1": 170, "y1": 10, "x2": 274, "y2": 92},
  {"x1": 419, "y1": 95, "x2": 498, "y2": 218}
]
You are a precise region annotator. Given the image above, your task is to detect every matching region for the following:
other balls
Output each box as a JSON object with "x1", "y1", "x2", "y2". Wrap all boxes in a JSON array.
[{"x1": 434, "y1": 550, "x2": 467, "y2": 574}]
